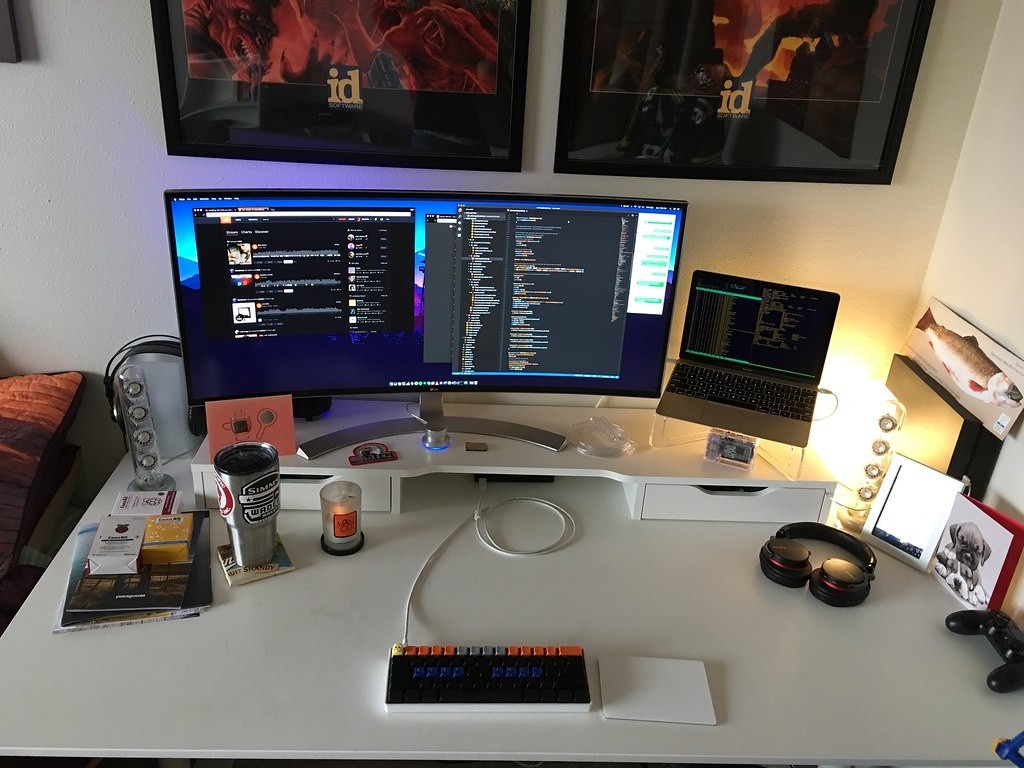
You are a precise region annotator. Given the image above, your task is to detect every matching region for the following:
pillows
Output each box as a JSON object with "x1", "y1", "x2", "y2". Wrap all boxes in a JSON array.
[{"x1": 0, "y1": 371, "x2": 85, "y2": 580}]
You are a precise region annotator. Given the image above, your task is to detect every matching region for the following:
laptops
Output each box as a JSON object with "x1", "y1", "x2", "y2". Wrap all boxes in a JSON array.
[{"x1": 656, "y1": 269, "x2": 840, "y2": 448}]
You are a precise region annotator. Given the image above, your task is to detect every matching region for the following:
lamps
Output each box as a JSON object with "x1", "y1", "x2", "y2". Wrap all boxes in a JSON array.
[
  {"x1": 836, "y1": 401, "x2": 906, "y2": 543},
  {"x1": 114, "y1": 365, "x2": 177, "y2": 492}
]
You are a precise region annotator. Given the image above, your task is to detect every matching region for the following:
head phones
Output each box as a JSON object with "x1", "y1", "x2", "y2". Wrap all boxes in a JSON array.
[
  {"x1": 103, "y1": 334, "x2": 208, "y2": 436},
  {"x1": 759, "y1": 522, "x2": 879, "y2": 607}
]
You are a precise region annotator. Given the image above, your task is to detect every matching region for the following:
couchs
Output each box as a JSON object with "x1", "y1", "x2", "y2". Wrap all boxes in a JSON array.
[{"x1": 0, "y1": 442, "x2": 86, "y2": 637}]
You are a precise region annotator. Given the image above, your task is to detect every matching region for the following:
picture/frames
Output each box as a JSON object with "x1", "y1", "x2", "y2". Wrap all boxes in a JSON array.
[
  {"x1": 857, "y1": 453, "x2": 968, "y2": 575},
  {"x1": 552, "y1": 0, "x2": 935, "y2": 185},
  {"x1": 150, "y1": 0, "x2": 531, "y2": 173}
]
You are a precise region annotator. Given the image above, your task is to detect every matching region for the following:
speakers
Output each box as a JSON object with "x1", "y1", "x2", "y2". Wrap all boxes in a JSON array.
[
  {"x1": 117, "y1": 364, "x2": 176, "y2": 492},
  {"x1": 836, "y1": 400, "x2": 906, "y2": 533}
]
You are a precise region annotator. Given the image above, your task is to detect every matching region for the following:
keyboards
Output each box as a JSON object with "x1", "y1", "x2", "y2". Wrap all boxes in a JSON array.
[{"x1": 385, "y1": 644, "x2": 593, "y2": 713}]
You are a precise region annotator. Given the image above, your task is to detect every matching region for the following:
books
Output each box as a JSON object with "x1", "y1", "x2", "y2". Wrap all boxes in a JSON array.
[{"x1": 51, "y1": 509, "x2": 215, "y2": 634}]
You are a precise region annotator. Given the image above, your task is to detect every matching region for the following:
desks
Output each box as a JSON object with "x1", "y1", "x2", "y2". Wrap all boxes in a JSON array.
[{"x1": 0, "y1": 397, "x2": 1024, "y2": 768}]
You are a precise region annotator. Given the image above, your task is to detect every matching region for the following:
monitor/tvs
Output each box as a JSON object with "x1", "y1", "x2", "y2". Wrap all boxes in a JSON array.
[{"x1": 164, "y1": 189, "x2": 690, "y2": 452}]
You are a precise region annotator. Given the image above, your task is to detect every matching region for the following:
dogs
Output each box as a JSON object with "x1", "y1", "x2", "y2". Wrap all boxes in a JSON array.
[{"x1": 935, "y1": 522, "x2": 992, "y2": 606}]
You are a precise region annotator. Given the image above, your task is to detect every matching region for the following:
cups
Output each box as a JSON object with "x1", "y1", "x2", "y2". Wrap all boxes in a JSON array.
[
  {"x1": 214, "y1": 442, "x2": 281, "y2": 567},
  {"x1": 321, "y1": 481, "x2": 364, "y2": 555}
]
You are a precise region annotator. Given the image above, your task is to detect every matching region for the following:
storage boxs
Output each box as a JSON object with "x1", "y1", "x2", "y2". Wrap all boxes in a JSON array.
[{"x1": 87, "y1": 491, "x2": 194, "y2": 575}]
